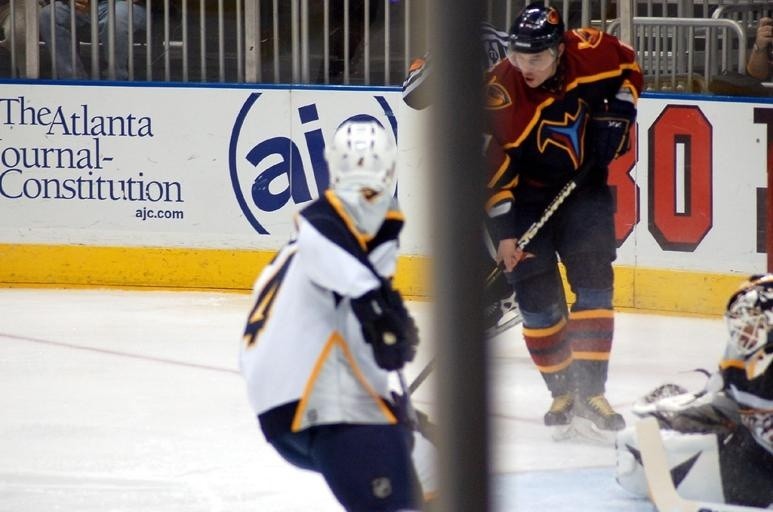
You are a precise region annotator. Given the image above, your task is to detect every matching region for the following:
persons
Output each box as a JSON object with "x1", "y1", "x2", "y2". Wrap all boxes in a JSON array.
[
  {"x1": 1, "y1": 0, "x2": 379, "y2": 85},
  {"x1": 401, "y1": 20, "x2": 519, "y2": 327},
  {"x1": 614, "y1": 274, "x2": 773, "y2": 512},
  {"x1": 746, "y1": 10, "x2": 773, "y2": 81},
  {"x1": 240, "y1": 120, "x2": 431, "y2": 512},
  {"x1": 482, "y1": 5, "x2": 646, "y2": 432}
]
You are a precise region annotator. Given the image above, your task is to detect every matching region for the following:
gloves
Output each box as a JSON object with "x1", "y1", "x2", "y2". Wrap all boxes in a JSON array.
[
  {"x1": 350, "y1": 278, "x2": 420, "y2": 373},
  {"x1": 585, "y1": 95, "x2": 639, "y2": 165}
]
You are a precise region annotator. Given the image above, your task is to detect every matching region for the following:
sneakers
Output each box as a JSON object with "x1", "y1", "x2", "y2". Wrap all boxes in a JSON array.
[
  {"x1": 544, "y1": 391, "x2": 574, "y2": 427},
  {"x1": 575, "y1": 394, "x2": 626, "y2": 433}
]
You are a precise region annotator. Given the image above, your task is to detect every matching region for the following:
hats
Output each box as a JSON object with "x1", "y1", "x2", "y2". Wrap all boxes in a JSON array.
[{"x1": 724, "y1": 274, "x2": 771, "y2": 379}]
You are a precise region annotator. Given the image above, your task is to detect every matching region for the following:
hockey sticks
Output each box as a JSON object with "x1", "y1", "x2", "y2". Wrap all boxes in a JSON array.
[
  {"x1": 383, "y1": 181, "x2": 578, "y2": 447},
  {"x1": 637, "y1": 417, "x2": 769, "y2": 512}
]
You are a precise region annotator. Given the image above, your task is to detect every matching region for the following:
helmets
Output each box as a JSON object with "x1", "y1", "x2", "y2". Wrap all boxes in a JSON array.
[
  {"x1": 330, "y1": 118, "x2": 399, "y2": 235},
  {"x1": 504, "y1": 4, "x2": 566, "y2": 89}
]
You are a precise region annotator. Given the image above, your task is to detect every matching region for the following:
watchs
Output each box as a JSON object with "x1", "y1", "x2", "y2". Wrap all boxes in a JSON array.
[{"x1": 753, "y1": 43, "x2": 768, "y2": 53}]
